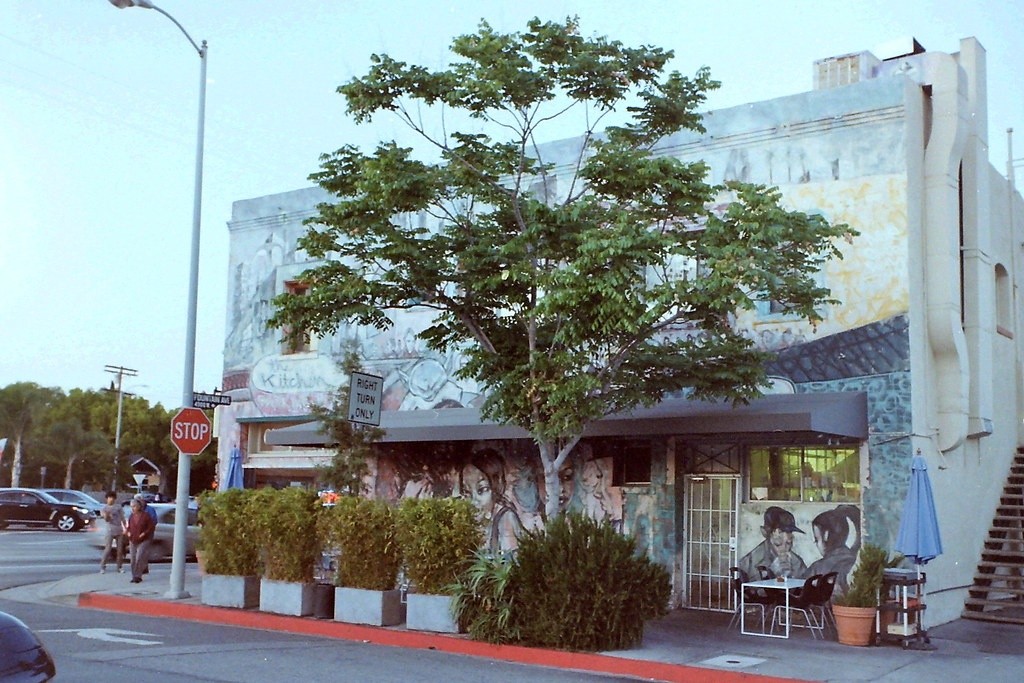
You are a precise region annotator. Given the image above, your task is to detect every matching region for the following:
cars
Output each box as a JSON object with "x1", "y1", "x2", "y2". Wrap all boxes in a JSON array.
[
  {"x1": 0, "y1": 487, "x2": 97, "y2": 533},
  {"x1": 87, "y1": 503, "x2": 203, "y2": 563},
  {"x1": 41, "y1": 488, "x2": 106, "y2": 519}
]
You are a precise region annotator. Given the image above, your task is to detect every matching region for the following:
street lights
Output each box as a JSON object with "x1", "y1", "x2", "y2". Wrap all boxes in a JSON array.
[{"x1": 107, "y1": 0, "x2": 213, "y2": 602}]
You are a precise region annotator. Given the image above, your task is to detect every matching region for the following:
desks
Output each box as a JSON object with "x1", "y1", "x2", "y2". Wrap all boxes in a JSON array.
[{"x1": 741, "y1": 579, "x2": 833, "y2": 639}]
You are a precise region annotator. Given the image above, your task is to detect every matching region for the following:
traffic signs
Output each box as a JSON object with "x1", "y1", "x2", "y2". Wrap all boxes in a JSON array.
[
  {"x1": 346, "y1": 371, "x2": 384, "y2": 426},
  {"x1": 193, "y1": 392, "x2": 232, "y2": 405},
  {"x1": 192, "y1": 401, "x2": 219, "y2": 409}
]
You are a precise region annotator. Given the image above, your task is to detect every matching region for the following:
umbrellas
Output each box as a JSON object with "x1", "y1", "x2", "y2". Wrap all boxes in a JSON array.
[
  {"x1": 220, "y1": 445, "x2": 246, "y2": 493},
  {"x1": 894, "y1": 447, "x2": 944, "y2": 653}
]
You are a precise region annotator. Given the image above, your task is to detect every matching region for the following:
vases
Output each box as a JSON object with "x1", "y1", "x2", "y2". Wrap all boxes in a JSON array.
[{"x1": 881, "y1": 597, "x2": 918, "y2": 641}]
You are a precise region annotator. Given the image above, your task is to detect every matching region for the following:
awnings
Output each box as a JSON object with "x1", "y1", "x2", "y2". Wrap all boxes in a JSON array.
[{"x1": 264, "y1": 392, "x2": 869, "y2": 454}]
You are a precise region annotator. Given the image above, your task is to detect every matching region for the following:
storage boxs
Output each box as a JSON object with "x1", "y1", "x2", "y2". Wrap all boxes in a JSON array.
[
  {"x1": 884, "y1": 568, "x2": 917, "y2": 580},
  {"x1": 888, "y1": 624, "x2": 918, "y2": 636}
]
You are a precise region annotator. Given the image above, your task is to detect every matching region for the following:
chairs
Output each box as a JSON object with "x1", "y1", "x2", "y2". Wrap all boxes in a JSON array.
[{"x1": 730, "y1": 567, "x2": 838, "y2": 640}]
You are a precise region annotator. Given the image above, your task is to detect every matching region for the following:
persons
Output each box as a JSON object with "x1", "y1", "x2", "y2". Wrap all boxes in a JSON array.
[{"x1": 98, "y1": 490, "x2": 159, "y2": 584}]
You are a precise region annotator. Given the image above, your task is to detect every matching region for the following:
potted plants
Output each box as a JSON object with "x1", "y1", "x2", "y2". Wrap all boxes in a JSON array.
[
  {"x1": 831, "y1": 544, "x2": 888, "y2": 646},
  {"x1": 193, "y1": 539, "x2": 207, "y2": 574},
  {"x1": 197, "y1": 485, "x2": 487, "y2": 633}
]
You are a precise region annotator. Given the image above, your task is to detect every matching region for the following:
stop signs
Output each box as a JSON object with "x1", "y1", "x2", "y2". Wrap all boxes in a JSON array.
[{"x1": 169, "y1": 406, "x2": 213, "y2": 456}]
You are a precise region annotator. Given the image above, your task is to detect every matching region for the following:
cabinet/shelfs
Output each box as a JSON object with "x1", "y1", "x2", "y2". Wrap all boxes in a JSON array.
[{"x1": 876, "y1": 572, "x2": 930, "y2": 649}]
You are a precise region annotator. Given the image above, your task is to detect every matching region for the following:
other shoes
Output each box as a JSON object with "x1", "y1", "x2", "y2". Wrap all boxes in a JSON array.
[
  {"x1": 101, "y1": 568, "x2": 105, "y2": 574},
  {"x1": 142, "y1": 570, "x2": 148, "y2": 574},
  {"x1": 118, "y1": 568, "x2": 125, "y2": 573},
  {"x1": 131, "y1": 578, "x2": 142, "y2": 583}
]
